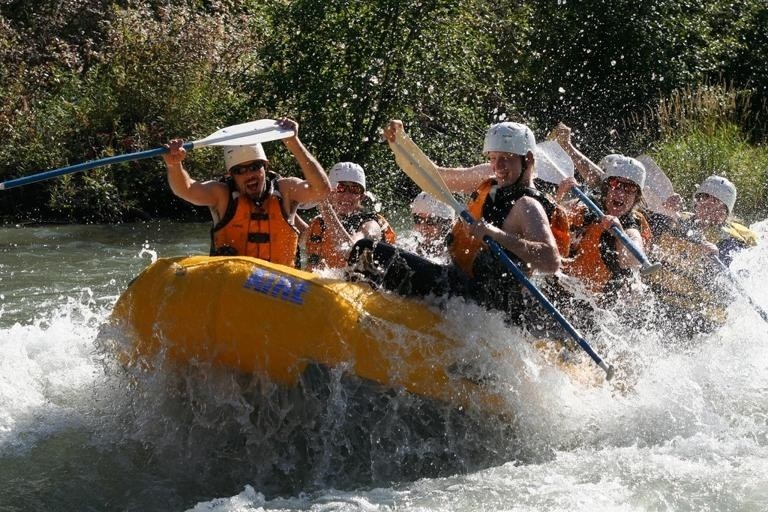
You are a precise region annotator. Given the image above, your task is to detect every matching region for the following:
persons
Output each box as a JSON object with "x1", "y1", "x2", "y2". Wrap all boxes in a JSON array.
[
  {"x1": 295, "y1": 117, "x2": 758, "y2": 341},
  {"x1": 161, "y1": 117, "x2": 331, "y2": 270}
]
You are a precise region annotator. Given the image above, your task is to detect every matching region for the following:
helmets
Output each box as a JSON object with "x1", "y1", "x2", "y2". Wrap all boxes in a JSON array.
[
  {"x1": 695, "y1": 175, "x2": 736, "y2": 213},
  {"x1": 482, "y1": 122, "x2": 537, "y2": 160},
  {"x1": 597, "y1": 154, "x2": 647, "y2": 190},
  {"x1": 224, "y1": 142, "x2": 269, "y2": 173},
  {"x1": 412, "y1": 190, "x2": 456, "y2": 220},
  {"x1": 329, "y1": 161, "x2": 366, "y2": 191}
]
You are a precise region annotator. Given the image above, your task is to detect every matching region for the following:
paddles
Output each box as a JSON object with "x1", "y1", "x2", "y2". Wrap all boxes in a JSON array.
[
  {"x1": 384, "y1": 125, "x2": 614, "y2": 380},
  {"x1": 636, "y1": 153, "x2": 764, "y2": 319},
  {"x1": 1, "y1": 117, "x2": 295, "y2": 192},
  {"x1": 535, "y1": 140, "x2": 651, "y2": 271}
]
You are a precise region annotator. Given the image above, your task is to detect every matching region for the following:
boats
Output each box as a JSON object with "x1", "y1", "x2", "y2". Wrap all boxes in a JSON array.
[{"x1": 101, "y1": 250, "x2": 733, "y2": 454}]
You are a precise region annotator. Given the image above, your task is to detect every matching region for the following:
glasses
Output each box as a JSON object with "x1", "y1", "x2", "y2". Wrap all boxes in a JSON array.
[
  {"x1": 414, "y1": 214, "x2": 442, "y2": 225},
  {"x1": 230, "y1": 160, "x2": 262, "y2": 175},
  {"x1": 607, "y1": 179, "x2": 639, "y2": 194},
  {"x1": 337, "y1": 184, "x2": 361, "y2": 194}
]
